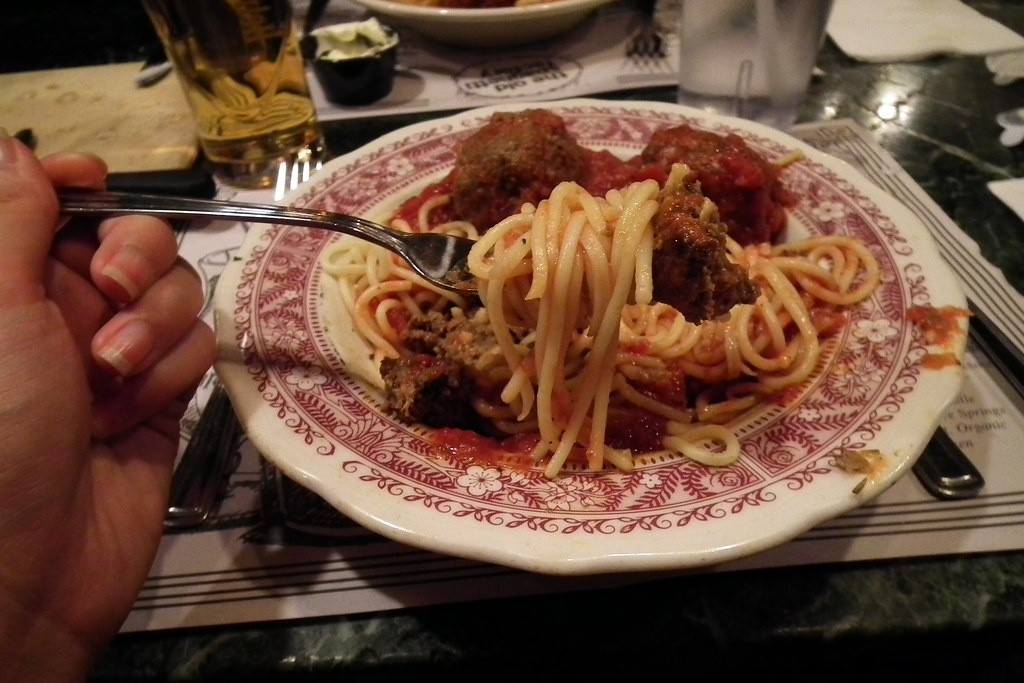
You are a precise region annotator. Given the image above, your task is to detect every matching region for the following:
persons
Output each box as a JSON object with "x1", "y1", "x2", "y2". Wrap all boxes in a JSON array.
[{"x1": 0, "y1": 125, "x2": 216, "y2": 683}]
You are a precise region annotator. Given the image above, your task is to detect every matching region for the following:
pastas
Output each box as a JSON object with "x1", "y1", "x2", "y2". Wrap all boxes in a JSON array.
[{"x1": 321, "y1": 160, "x2": 880, "y2": 479}]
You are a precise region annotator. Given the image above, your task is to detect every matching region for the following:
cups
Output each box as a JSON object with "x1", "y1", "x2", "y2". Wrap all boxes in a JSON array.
[
  {"x1": 142, "y1": 1, "x2": 330, "y2": 189},
  {"x1": 678, "y1": 1, "x2": 833, "y2": 133},
  {"x1": 310, "y1": 26, "x2": 399, "y2": 107}
]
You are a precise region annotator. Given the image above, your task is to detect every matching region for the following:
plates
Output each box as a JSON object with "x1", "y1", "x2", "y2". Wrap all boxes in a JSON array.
[
  {"x1": 214, "y1": 98, "x2": 969, "y2": 575},
  {"x1": 353, "y1": 0, "x2": 613, "y2": 50}
]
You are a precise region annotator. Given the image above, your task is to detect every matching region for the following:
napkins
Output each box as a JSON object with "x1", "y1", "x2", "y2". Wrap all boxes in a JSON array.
[{"x1": 826, "y1": 0, "x2": 1024, "y2": 66}]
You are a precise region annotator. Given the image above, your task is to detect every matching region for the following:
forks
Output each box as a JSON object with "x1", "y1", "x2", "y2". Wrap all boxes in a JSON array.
[
  {"x1": 253, "y1": 161, "x2": 323, "y2": 553},
  {"x1": 59, "y1": 190, "x2": 480, "y2": 294}
]
